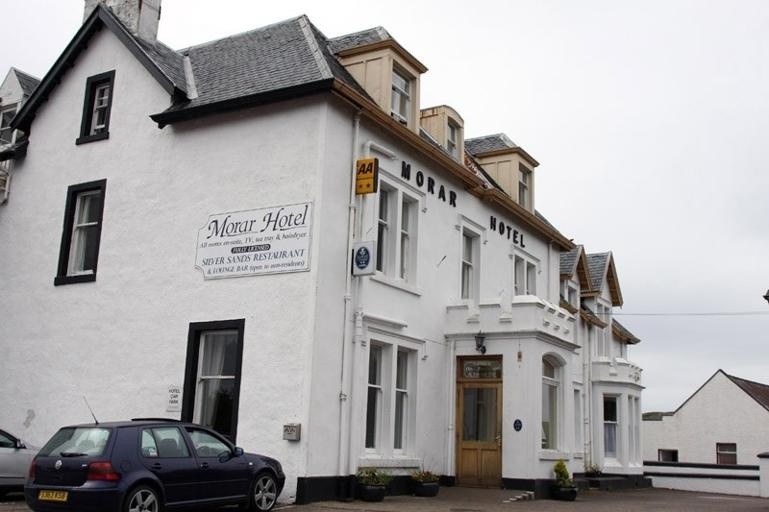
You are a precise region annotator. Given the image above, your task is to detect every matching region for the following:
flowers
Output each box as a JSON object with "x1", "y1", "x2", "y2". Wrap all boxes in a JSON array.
[{"x1": 410, "y1": 464, "x2": 442, "y2": 481}]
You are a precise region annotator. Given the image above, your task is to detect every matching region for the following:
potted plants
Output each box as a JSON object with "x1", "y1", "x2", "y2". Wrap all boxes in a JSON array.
[
  {"x1": 553, "y1": 460, "x2": 577, "y2": 500},
  {"x1": 357, "y1": 462, "x2": 393, "y2": 501}
]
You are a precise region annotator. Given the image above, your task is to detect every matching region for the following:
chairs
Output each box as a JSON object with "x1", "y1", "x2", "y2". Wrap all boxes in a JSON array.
[{"x1": 159, "y1": 438, "x2": 179, "y2": 459}]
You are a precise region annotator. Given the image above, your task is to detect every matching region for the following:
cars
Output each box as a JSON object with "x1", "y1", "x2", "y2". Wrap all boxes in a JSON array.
[
  {"x1": 25, "y1": 395, "x2": 286, "y2": 509},
  {"x1": 1, "y1": 429, "x2": 44, "y2": 490}
]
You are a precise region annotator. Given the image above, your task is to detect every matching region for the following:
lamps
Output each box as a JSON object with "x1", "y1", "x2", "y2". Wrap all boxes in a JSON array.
[{"x1": 473, "y1": 329, "x2": 489, "y2": 354}]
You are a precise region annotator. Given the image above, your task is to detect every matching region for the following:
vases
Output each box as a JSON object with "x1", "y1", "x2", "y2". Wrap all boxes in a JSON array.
[{"x1": 417, "y1": 482, "x2": 439, "y2": 497}]
